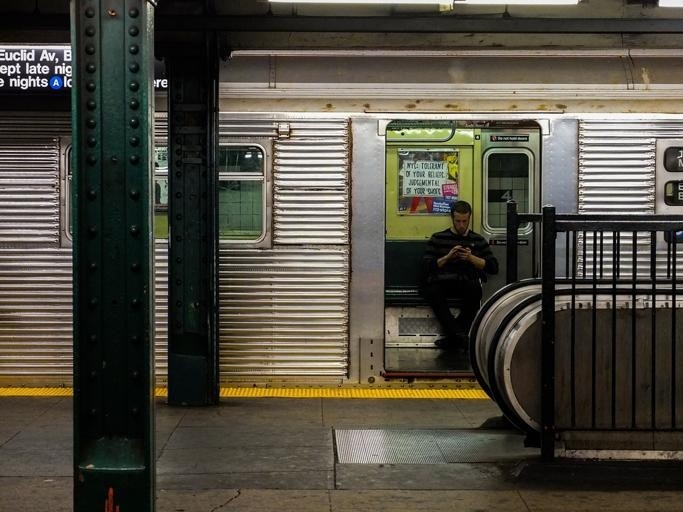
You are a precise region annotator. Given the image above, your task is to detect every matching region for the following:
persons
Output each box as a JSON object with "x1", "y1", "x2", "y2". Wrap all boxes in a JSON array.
[{"x1": 420, "y1": 201, "x2": 499, "y2": 350}]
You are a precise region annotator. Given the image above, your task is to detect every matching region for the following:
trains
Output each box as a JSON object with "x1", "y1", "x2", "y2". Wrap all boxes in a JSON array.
[{"x1": 0, "y1": 74, "x2": 683, "y2": 390}]
